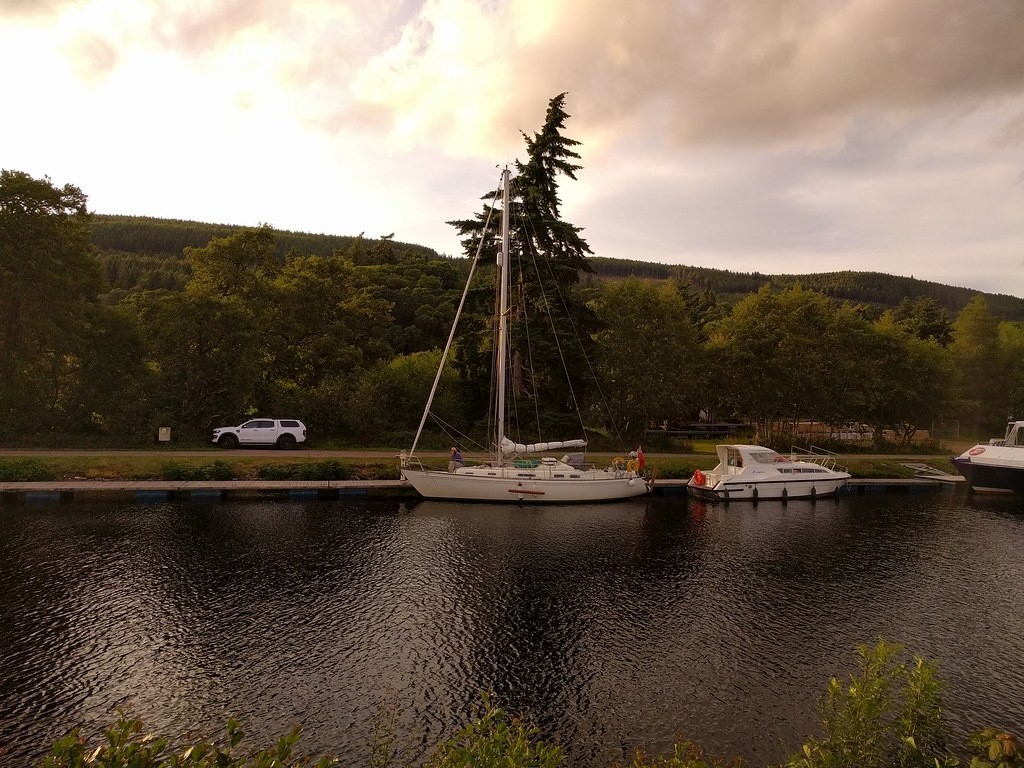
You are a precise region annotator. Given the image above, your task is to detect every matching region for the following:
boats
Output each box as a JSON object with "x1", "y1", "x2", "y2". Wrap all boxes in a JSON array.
[
  {"x1": 949, "y1": 419, "x2": 1024, "y2": 496},
  {"x1": 685, "y1": 444, "x2": 854, "y2": 504}
]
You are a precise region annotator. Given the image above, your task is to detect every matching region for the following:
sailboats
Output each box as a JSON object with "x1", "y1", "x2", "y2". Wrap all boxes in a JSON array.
[{"x1": 394, "y1": 160, "x2": 654, "y2": 507}]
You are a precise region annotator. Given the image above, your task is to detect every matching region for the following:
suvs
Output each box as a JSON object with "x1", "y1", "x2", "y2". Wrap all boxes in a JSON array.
[{"x1": 211, "y1": 416, "x2": 308, "y2": 451}]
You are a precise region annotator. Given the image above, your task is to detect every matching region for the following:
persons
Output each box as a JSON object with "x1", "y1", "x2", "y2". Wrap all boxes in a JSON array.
[
  {"x1": 637, "y1": 444, "x2": 644, "y2": 471},
  {"x1": 448, "y1": 447, "x2": 464, "y2": 474}
]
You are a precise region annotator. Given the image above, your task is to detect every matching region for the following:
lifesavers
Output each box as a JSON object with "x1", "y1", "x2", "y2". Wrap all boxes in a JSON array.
[
  {"x1": 611, "y1": 457, "x2": 625, "y2": 467},
  {"x1": 626, "y1": 459, "x2": 640, "y2": 472},
  {"x1": 694, "y1": 469, "x2": 706, "y2": 486},
  {"x1": 969, "y1": 447, "x2": 985, "y2": 456}
]
